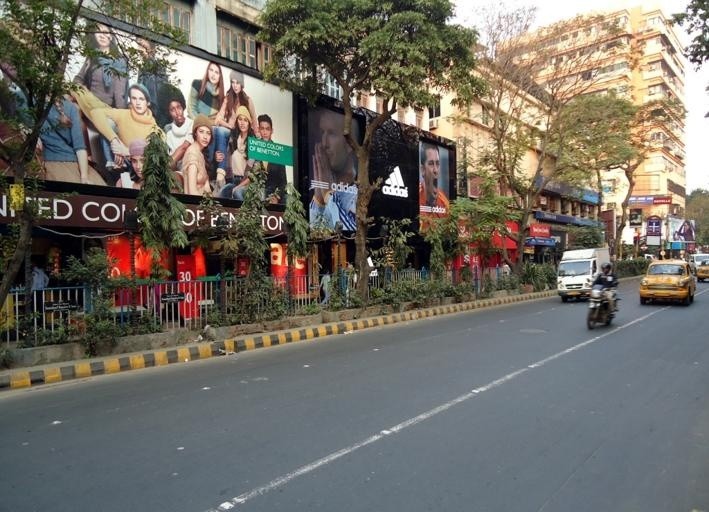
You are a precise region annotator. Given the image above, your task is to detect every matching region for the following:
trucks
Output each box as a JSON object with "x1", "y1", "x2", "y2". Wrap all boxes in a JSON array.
[{"x1": 556, "y1": 248, "x2": 612, "y2": 304}]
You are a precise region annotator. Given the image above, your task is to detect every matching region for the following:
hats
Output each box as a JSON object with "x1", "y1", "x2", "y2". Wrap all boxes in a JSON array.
[
  {"x1": 130, "y1": 140, "x2": 146, "y2": 156},
  {"x1": 230, "y1": 70, "x2": 244, "y2": 87},
  {"x1": 236, "y1": 106, "x2": 251, "y2": 122},
  {"x1": 193, "y1": 114, "x2": 212, "y2": 131}
]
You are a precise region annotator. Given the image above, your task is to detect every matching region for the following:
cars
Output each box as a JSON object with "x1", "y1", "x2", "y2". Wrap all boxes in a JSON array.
[{"x1": 639, "y1": 253, "x2": 709, "y2": 307}]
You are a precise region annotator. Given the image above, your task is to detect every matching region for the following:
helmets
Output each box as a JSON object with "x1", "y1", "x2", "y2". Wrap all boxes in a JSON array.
[{"x1": 602, "y1": 263, "x2": 610, "y2": 274}]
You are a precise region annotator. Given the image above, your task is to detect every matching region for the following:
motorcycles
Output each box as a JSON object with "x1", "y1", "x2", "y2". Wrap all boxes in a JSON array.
[{"x1": 586, "y1": 276, "x2": 622, "y2": 330}]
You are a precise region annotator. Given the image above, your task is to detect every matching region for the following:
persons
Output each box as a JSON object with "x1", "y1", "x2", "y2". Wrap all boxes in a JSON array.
[
  {"x1": 592, "y1": 262, "x2": 617, "y2": 315},
  {"x1": 182, "y1": 112, "x2": 213, "y2": 197},
  {"x1": 678, "y1": 220, "x2": 696, "y2": 242},
  {"x1": 310, "y1": 108, "x2": 359, "y2": 235},
  {"x1": 419, "y1": 142, "x2": 451, "y2": 232},
  {"x1": 346, "y1": 266, "x2": 357, "y2": 307},
  {"x1": 187, "y1": 62, "x2": 288, "y2": 205},
  {"x1": 0, "y1": 14, "x2": 193, "y2": 194},
  {"x1": 320, "y1": 269, "x2": 333, "y2": 304},
  {"x1": 502, "y1": 261, "x2": 510, "y2": 274},
  {"x1": 29, "y1": 263, "x2": 48, "y2": 314}
]
